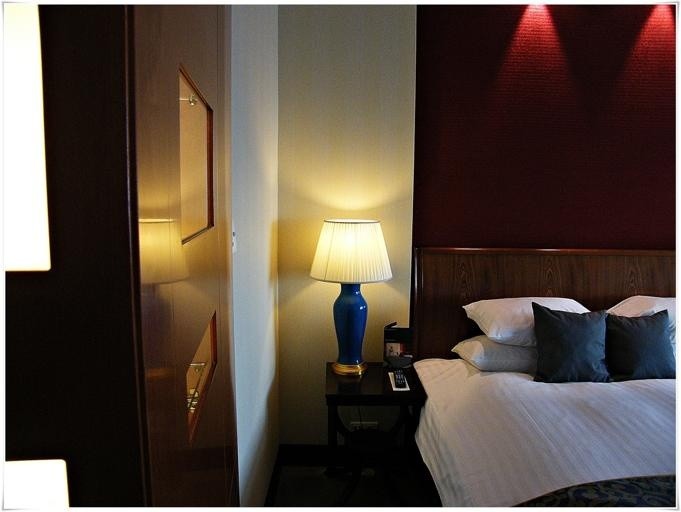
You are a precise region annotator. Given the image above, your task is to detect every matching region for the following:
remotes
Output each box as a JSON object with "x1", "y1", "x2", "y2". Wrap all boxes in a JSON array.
[{"x1": 394, "y1": 369, "x2": 406, "y2": 388}]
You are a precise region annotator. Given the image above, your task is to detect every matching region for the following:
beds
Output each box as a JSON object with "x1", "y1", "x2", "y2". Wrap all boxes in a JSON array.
[{"x1": 411, "y1": 239, "x2": 675, "y2": 507}]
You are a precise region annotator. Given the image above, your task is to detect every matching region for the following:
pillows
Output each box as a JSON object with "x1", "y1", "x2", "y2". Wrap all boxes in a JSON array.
[{"x1": 457, "y1": 282, "x2": 674, "y2": 383}]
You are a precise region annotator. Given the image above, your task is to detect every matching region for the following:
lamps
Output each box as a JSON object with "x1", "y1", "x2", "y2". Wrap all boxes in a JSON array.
[{"x1": 309, "y1": 219, "x2": 391, "y2": 374}]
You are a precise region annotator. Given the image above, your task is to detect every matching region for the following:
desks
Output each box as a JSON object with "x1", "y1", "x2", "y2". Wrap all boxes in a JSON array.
[{"x1": 325, "y1": 360, "x2": 423, "y2": 507}]
